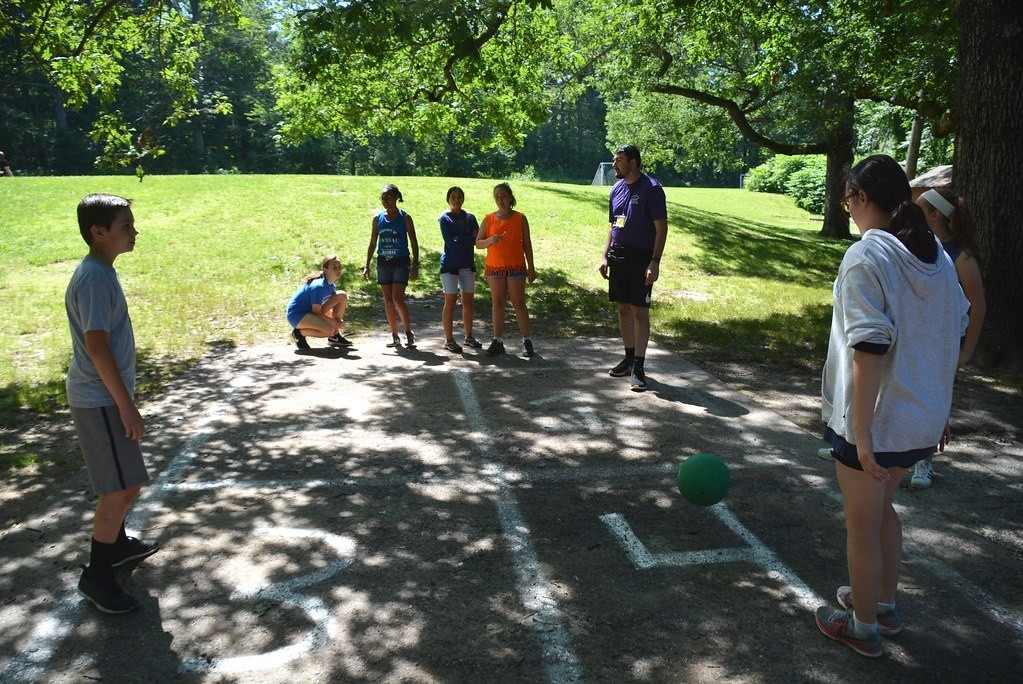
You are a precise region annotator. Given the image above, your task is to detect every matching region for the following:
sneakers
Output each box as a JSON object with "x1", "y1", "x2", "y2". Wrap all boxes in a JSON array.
[
  {"x1": 77, "y1": 564, "x2": 134, "y2": 613},
  {"x1": 912, "y1": 460, "x2": 932, "y2": 487},
  {"x1": 522, "y1": 339, "x2": 533, "y2": 356},
  {"x1": 444, "y1": 339, "x2": 462, "y2": 352},
  {"x1": 815, "y1": 605, "x2": 882, "y2": 657},
  {"x1": 464, "y1": 336, "x2": 482, "y2": 348},
  {"x1": 292, "y1": 330, "x2": 311, "y2": 350},
  {"x1": 818, "y1": 448, "x2": 836, "y2": 462},
  {"x1": 631, "y1": 368, "x2": 647, "y2": 391},
  {"x1": 112, "y1": 536, "x2": 159, "y2": 566},
  {"x1": 328, "y1": 333, "x2": 352, "y2": 345},
  {"x1": 485, "y1": 339, "x2": 505, "y2": 356},
  {"x1": 836, "y1": 586, "x2": 902, "y2": 636},
  {"x1": 609, "y1": 359, "x2": 633, "y2": 375}
]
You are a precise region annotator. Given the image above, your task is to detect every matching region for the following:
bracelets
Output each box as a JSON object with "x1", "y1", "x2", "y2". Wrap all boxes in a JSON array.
[
  {"x1": 651, "y1": 256, "x2": 660, "y2": 263},
  {"x1": 412, "y1": 262, "x2": 420, "y2": 265}
]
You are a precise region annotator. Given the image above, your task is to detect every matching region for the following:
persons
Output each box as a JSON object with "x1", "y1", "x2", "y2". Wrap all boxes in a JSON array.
[
  {"x1": 915, "y1": 188, "x2": 986, "y2": 370},
  {"x1": 598, "y1": 144, "x2": 668, "y2": 391},
  {"x1": 286, "y1": 255, "x2": 352, "y2": 351},
  {"x1": 476, "y1": 182, "x2": 536, "y2": 357},
  {"x1": 65, "y1": 193, "x2": 160, "y2": 615},
  {"x1": 438, "y1": 186, "x2": 482, "y2": 352},
  {"x1": 363, "y1": 183, "x2": 420, "y2": 347},
  {"x1": 815, "y1": 154, "x2": 971, "y2": 657},
  {"x1": 0, "y1": 151, "x2": 13, "y2": 176}
]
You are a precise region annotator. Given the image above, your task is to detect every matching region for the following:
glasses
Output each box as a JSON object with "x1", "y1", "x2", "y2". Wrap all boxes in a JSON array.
[
  {"x1": 840, "y1": 188, "x2": 861, "y2": 213},
  {"x1": 379, "y1": 195, "x2": 395, "y2": 200}
]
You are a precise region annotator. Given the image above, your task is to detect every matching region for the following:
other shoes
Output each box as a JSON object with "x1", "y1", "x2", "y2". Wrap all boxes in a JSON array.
[
  {"x1": 405, "y1": 330, "x2": 415, "y2": 346},
  {"x1": 387, "y1": 337, "x2": 400, "y2": 346}
]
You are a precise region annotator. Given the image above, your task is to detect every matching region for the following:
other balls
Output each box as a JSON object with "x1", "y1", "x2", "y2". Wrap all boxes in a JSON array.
[{"x1": 677, "y1": 453, "x2": 730, "y2": 506}]
